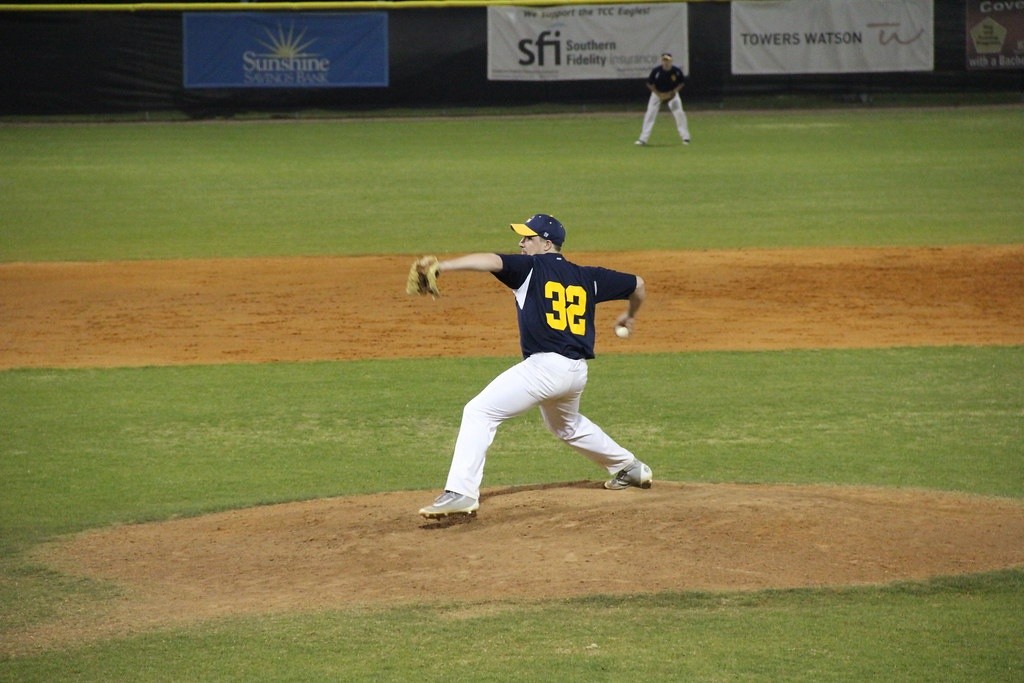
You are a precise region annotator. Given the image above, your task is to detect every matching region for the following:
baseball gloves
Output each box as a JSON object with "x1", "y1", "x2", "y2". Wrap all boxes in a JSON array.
[{"x1": 405, "y1": 252, "x2": 442, "y2": 301}]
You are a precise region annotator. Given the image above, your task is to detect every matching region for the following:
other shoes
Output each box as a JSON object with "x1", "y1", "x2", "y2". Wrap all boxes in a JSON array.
[
  {"x1": 634, "y1": 140, "x2": 645, "y2": 145},
  {"x1": 683, "y1": 140, "x2": 689, "y2": 145}
]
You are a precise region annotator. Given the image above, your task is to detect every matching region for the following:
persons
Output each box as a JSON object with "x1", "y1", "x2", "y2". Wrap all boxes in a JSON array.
[
  {"x1": 635, "y1": 52, "x2": 692, "y2": 146},
  {"x1": 417, "y1": 217, "x2": 652, "y2": 519}
]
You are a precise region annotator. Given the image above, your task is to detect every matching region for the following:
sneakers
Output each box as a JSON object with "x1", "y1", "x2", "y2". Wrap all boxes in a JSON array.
[
  {"x1": 419, "y1": 491, "x2": 480, "y2": 518},
  {"x1": 603, "y1": 461, "x2": 654, "y2": 490}
]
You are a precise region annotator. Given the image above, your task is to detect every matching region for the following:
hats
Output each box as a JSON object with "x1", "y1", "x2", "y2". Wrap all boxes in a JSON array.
[
  {"x1": 510, "y1": 214, "x2": 566, "y2": 247},
  {"x1": 661, "y1": 51, "x2": 672, "y2": 60}
]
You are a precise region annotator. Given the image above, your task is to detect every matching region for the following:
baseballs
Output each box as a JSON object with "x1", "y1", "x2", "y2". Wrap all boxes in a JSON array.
[{"x1": 615, "y1": 328, "x2": 630, "y2": 341}]
